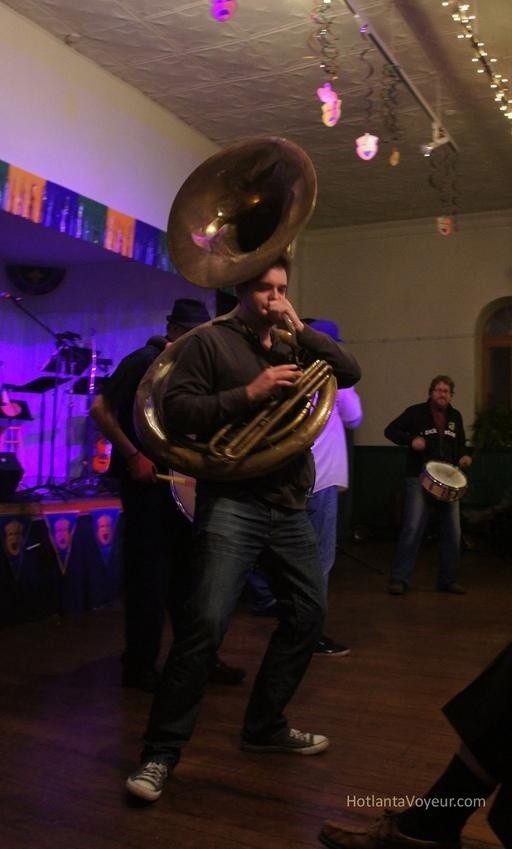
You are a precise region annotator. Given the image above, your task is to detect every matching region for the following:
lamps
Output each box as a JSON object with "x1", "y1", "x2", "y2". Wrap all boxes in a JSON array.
[
  {"x1": 309, "y1": 0, "x2": 342, "y2": 129},
  {"x1": 208, "y1": 0, "x2": 238, "y2": 22},
  {"x1": 358, "y1": 21, "x2": 459, "y2": 238}
]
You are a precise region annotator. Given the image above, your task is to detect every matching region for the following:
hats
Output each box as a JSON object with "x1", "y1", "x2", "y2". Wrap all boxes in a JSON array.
[{"x1": 163, "y1": 298, "x2": 212, "y2": 330}]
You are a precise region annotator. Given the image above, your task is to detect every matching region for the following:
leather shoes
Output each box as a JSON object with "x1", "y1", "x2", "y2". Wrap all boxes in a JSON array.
[
  {"x1": 389, "y1": 584, "x2": 407, "y2": 595},
  {"x1": 317, "y1": 805, "x2": 463, "y2": 849},
  {"x1": 435, "y1": 575, "x2": 467, "y2": 595}
]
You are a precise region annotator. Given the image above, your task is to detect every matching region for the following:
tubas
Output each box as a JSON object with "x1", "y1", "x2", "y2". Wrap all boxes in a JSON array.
[{"x1": 133, "y1": 136, "x2": 337, "y2": 479}]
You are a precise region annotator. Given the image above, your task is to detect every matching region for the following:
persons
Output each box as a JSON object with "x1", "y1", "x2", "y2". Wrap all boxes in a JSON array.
[
  {"x1": 296, "y1": 320, "x2": 367, "y2": 660},
  {"x1": 111, "y1": 256, "x2": 360, "y2": 803},
  {"x1": 383, "y1": 373, "x2": 470, "y2": 597},
  {"x1": 314, "y1": 634, "x2": 512, "y2": 849},
  {"x1": 90, "y1": 295, "x2": 249, "y2": 694}
]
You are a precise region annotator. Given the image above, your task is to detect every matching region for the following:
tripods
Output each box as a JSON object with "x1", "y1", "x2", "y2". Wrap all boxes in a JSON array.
[{"x1": 8, "y1": 360, "x2": 115, "y2": 501}]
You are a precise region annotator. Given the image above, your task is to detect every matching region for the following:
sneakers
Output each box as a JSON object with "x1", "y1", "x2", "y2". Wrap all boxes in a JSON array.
[
  {"x1": 207, "y1": 655, "x2": 250, "y2": 685},
  {"x1": 241, "y1": 726, "x2": 333, "y2": 755},
  {"x1": 121, "y1": 652, "x2": 164, "y2": 692},
  {"x1": 121, "y1": 759, "x2": 172, "y2": 807},
  {"x1": 312, "y1": 635, "x2": 355, "y2": 659}
]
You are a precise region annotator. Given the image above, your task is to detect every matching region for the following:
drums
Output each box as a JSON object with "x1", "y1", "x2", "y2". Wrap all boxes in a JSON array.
[
  {"x1": 419, "y1": 460, "x2": 469, "y2": 502},
  {"x1": 168, "y1": 466, "x2": 197, "y2": 523}
]
